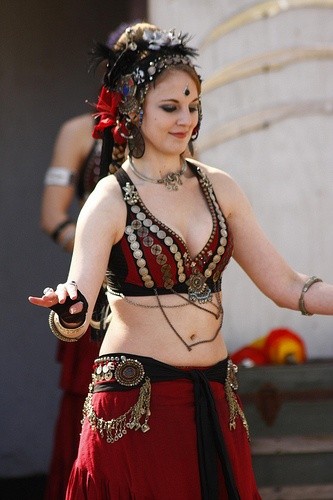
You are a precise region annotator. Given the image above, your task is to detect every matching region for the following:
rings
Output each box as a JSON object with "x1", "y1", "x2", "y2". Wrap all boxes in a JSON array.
[{"x1": 68, "y1": 280, "x2": 76, "y2": 287}]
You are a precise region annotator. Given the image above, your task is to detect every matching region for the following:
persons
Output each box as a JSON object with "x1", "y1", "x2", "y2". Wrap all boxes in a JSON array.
[
  {"x1": 27, "y1": 24, "x2": 332, "y2": 500},
  {"x1": 39, "y1": 111, "x2": 111, "y2": 500}
]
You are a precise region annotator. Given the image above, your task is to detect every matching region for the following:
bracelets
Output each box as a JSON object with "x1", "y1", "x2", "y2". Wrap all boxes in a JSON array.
[{"x1": 297, "y1": 275, "x2": 322, "y2": 317}]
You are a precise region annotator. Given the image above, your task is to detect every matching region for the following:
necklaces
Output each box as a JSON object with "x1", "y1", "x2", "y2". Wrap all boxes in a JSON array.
[{"x1": 126, "y1": 144, "x2": 193, "y2": 193}]
